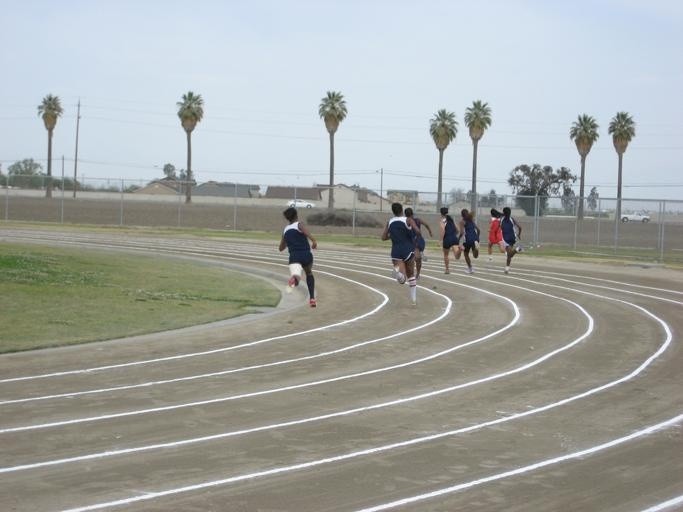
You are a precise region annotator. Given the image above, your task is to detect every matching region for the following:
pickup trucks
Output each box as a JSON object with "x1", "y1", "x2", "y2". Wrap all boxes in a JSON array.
[{"x1": 620, "y1": 210, "x2": 651, "y2": 224}]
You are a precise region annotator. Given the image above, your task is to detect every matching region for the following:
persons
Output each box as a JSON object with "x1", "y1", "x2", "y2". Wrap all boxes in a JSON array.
[
  {"x1": 437, "y1": 208, "x2": 465, "y2": 274},
  {"x1": 495, "y1": 208, "x2": 524, "y2": 272},
  {"x1": 279, "y1": 208, "x2": 317, "y2": 307},
  {"x1": 488, "y1": 209, "x2": 507, "y2": 262},
  {"x1": 459, "y1": 210, "x2": 480, "y2": 274},
  {"x1": 382, "y1": 203, "x2": 432, "y2": 306}
]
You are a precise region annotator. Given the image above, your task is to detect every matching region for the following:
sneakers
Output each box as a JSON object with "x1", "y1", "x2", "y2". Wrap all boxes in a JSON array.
[
  {"x1": 309, "y1": 298, "x2": 316, "y2": 307},
  {"x1": 458, "y1": 246, "x2": 464, "y2": 250},
  {"x1": 288, "y1": 277, "x2": 298, "y2": 288}
]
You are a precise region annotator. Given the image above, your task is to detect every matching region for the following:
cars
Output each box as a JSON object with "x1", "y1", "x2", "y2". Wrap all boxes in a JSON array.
[{"x1": 287, "y1": 199, "x2": 316, "y2": 209}]
